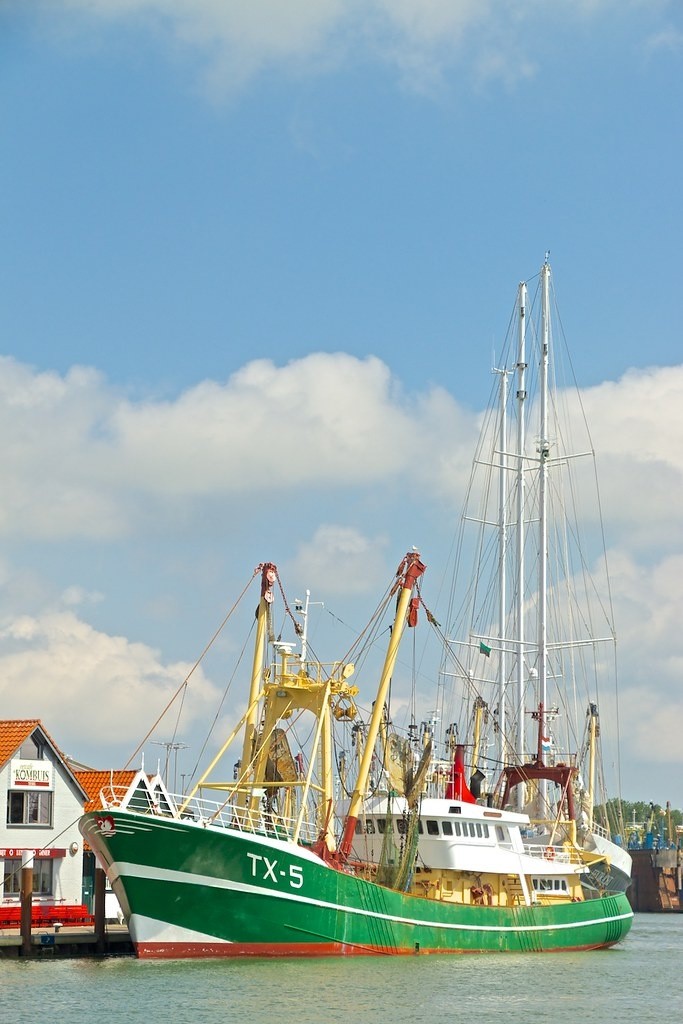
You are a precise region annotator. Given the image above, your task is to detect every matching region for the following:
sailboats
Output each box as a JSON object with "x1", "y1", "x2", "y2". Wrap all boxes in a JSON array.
[{"x1": 436, "y1": 247, "x2": 636, "y2": 897}]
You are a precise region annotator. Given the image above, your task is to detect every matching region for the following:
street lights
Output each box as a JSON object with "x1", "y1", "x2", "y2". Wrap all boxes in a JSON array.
[{"x1": 150, "y1": 741, "x2": 190, "y2": 804}]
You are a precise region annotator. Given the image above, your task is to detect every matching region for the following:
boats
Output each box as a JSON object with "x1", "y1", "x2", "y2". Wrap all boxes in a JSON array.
[{"x1": 77, "y1": 544, "x2": 634, "y2": 963}]
